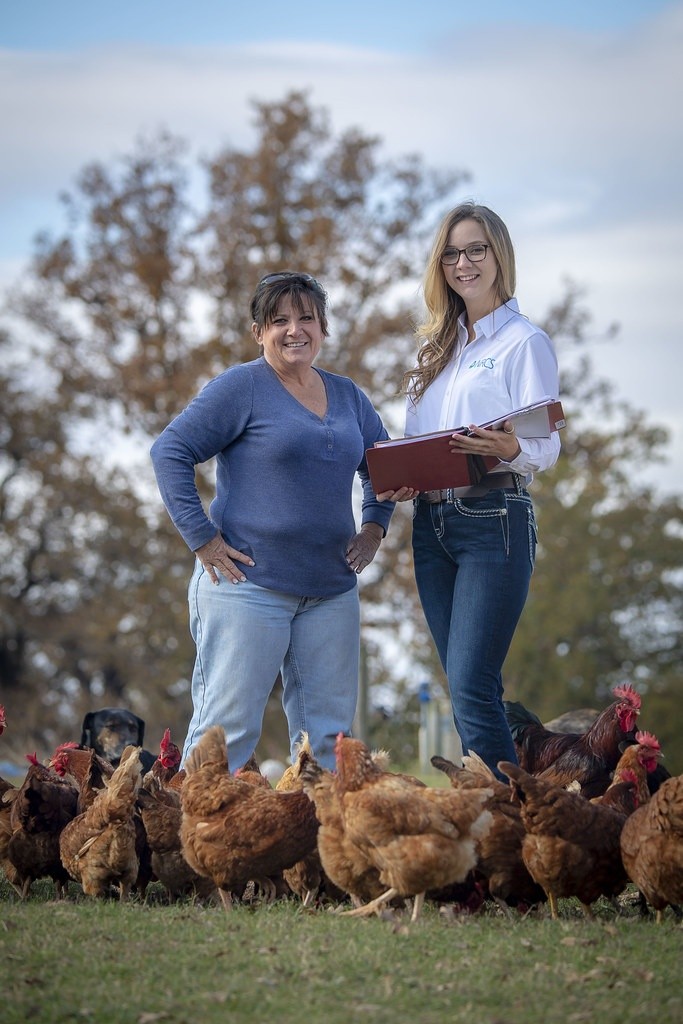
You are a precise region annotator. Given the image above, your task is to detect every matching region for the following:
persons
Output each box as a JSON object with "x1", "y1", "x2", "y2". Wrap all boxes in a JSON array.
[
  {"x1": 149, "y1": 271, "x2": 396, "y2": 775},
  {"x1": 375, "y1": 198, "x2": 562, "y2": 785}
]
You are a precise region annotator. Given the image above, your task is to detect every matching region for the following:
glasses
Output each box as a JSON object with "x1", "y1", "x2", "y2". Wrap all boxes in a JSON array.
[
  {"x1": 259, "y1": 272, "x2": 314, "y2": 286},
  {"x1": 438, "y1": 243, "x2": 492, "y2": 266}
]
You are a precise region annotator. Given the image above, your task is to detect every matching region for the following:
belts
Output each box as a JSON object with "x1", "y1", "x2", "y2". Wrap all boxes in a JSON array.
[{"x1": 419, "y1": 471, "x2": 528, "y2": 503}]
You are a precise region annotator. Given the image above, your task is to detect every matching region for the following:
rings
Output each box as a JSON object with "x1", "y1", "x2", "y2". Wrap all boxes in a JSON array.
[{"x1": 504, "y1": 426, "x2": 515, "y2": 434}]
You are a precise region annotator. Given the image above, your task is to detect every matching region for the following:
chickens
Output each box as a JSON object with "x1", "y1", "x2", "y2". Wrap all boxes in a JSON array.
[{"x1": 0, "y1": 683, "x2": 683, "y2": 924}]
